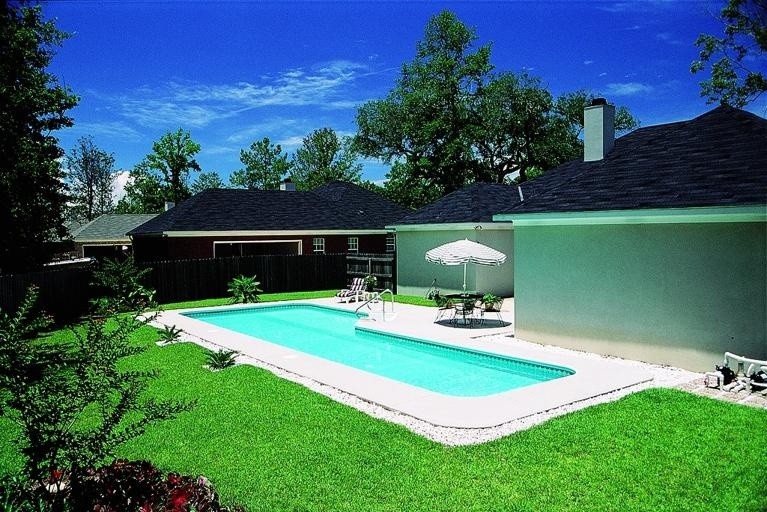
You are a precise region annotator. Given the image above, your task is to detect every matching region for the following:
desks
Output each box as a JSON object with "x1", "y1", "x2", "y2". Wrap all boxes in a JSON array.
[{"x1": 363, "y1": 292, "x2": 378, "y2": 304}]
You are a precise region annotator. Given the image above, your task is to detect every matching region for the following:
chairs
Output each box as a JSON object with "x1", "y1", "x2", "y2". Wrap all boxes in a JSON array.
[
  {"x1": 333, "y1": 277, "x2": 367, "y2": 304},
  {"x1": 432, "y1": 290, "x2": 506, "y2": 328}
]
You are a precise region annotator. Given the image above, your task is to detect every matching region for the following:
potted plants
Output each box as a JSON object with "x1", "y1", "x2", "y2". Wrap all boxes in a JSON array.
[
  {"x1": 226, "y1": 273, "x2": 263, "y2": 304},
  {"x1": 365, "y1": 275, "x2": 378, "y2": 292}
]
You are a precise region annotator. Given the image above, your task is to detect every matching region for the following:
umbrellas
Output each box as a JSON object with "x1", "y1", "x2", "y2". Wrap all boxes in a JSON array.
[{"x1": 424, "y1": 238, "x2": 508, "y2": 291}]
